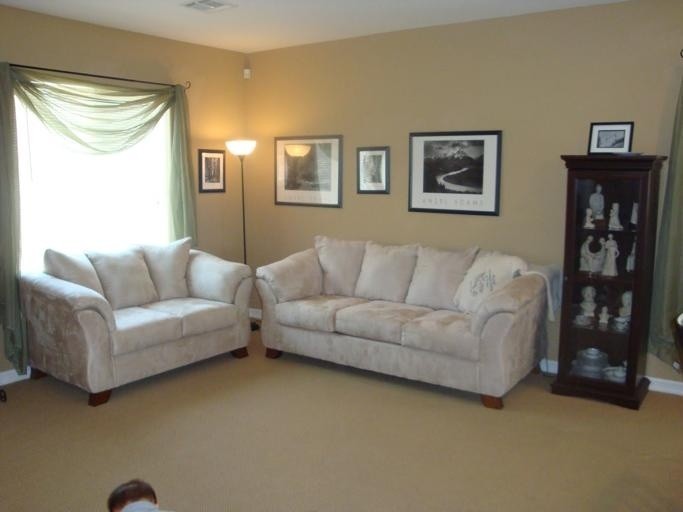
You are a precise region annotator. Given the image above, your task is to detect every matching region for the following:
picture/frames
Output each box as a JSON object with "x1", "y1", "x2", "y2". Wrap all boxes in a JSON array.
[
  {"x1": 198, "y1": 149, "x2": 223, "y2": 191},
  {"x1": 355, "y1": 144, "x2": 390, "y2": 193},
  {"x1": 408, "y1": 130, "x2": 504, "y2": 216},
  {"x1": 587, "y1": 122, "x2": 634, "y2": 156},
  {"x1": 272, "y1": 136, "x2": 344, "y2": 208}
]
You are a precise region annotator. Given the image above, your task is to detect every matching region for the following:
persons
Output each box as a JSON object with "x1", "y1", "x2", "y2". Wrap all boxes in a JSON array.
[
  {"x1": 105, "y1": 478, "x2": 162, "y2": 512},
  {"x1": 578, "y1": 182, "x2": 638, "y2": 334}
]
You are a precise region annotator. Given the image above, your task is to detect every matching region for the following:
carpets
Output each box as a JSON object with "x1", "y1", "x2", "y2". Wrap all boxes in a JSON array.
[{"x1": 1, "y1": 372, "x2": 682, "y2": 511}]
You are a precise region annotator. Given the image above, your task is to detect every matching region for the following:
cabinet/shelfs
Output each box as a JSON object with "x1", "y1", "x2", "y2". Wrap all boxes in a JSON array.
[{"x1": 551, "y1": 152, "x2": 667, "y2": 410}]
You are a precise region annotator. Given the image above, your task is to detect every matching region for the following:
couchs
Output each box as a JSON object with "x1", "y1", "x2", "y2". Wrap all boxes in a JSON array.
[
  {"x1": 19, "y1": 237, "x2": 256, "y2": 404},
  {"x1": 255, "y1": 236, "x2": 550, "y2": 411}
]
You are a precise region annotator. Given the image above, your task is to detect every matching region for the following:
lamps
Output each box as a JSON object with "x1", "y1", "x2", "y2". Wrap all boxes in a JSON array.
[{"x1": 224, "y1": 139, "x2": 260, "y2": 267}]
